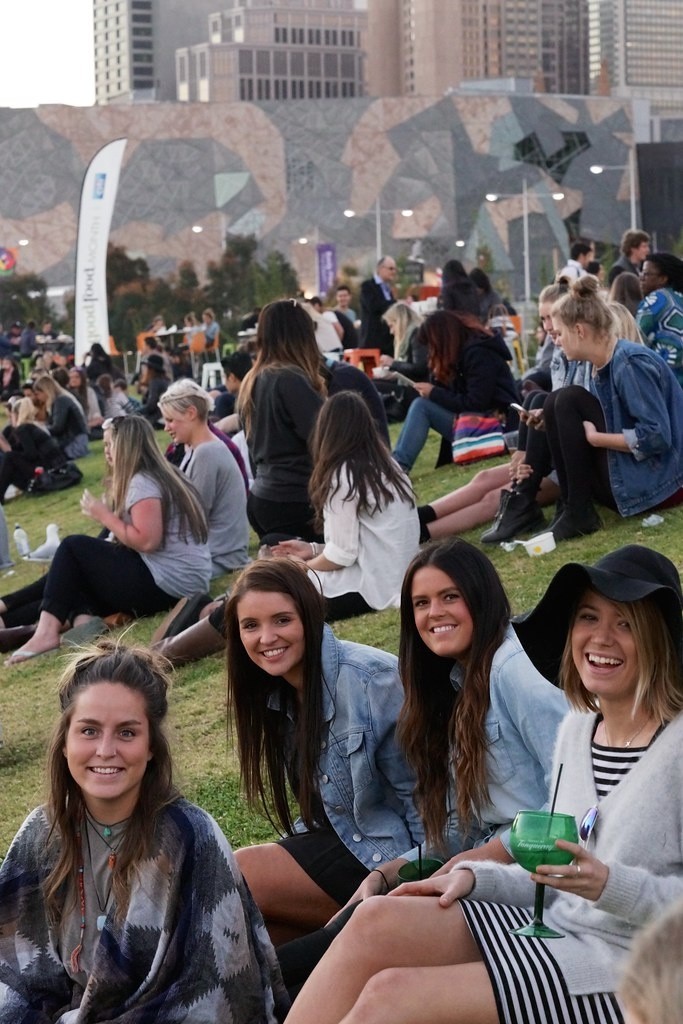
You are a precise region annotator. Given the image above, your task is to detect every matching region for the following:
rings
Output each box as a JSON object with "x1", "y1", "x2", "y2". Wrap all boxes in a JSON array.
[{"x1": 574, "y1": 865, "x2": 580, "y2": 879}]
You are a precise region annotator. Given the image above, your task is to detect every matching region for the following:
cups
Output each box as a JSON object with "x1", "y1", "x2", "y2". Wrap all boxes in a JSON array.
[
  {"x1": 397, "y1": 858, "x2": 445, "y2": 889},
  {"x1": 502, "y1": 430, "x2": 519, "y2": 456}
]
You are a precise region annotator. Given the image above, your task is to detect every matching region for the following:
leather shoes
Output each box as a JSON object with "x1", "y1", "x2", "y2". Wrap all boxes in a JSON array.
[
  {"x1": 530, "y1": 498, "x2": 603, "y2": 544},
  {"x1": 480, "y1": 487, "x2": 547, "y2": 544}
]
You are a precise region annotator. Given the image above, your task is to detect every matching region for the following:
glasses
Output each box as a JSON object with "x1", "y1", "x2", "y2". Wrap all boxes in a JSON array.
[
  {"x1": 289, "y1": 299, "x2": 298, "y2": 309},
  {"x1": 639, "y1": 272, "x2": 660, "y2": 280},
  {"x1": 383, "y1": 266, "x2": 395, "y2": 271},
  {"x1": 110, "y1": 416, "x2": 124, "y2": 434}
]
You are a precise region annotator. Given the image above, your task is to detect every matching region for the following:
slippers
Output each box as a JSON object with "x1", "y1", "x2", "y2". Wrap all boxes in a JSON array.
[
  {"x1": 5, "y1": 647, "x2": 59, "y2": 666},
  {"x1": 59, "y1": 615, "x2": 107, "y2": 646}
]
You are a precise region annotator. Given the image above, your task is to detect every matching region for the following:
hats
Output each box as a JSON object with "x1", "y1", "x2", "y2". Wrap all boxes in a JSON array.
[
  {"x1": 140, "y1": 355, "x2": 167, "y2": 373},
  {"x1": 509, "y1": 543, "x2": 683, "y2": 692}
]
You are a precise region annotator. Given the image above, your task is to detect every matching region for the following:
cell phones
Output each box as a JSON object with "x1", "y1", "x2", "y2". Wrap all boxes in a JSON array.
[{"x1": 510, "y1": 403, "x2": 538, "y2": 422}]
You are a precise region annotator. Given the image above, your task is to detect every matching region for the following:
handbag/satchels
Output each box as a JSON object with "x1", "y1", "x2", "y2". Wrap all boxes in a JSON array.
[
  {"x1": 374, "y1": 380, "x2": 408, "y2": 422},
  {"x1": 29, "y1": 464, "x2": 83, "y2": 496},
  {"x1": 450, "y1": 412, "x2": 506, "y2": 464}
]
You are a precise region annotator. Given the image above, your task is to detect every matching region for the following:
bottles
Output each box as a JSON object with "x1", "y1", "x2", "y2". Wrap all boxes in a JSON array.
[
  {"x1": 33, "y1": 467, "x2": 43, "y2": 480},
  {"x1": 13, "y1": 523, "x2": 30, "y2": 556}
]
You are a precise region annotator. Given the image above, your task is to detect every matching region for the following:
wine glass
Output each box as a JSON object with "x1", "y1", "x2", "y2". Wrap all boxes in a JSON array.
[{"x1": 506, "y1": 810, "x2": 579, "y2": 939}]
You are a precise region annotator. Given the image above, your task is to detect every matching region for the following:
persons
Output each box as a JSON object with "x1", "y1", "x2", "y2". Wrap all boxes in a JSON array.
[{"x1": 0, "y1": 228, "x2": 683, "y2": 1024}]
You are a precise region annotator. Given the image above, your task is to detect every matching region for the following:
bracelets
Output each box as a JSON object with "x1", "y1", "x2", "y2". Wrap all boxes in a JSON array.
[
  {"x1": 310, "y1": 543, "x2": 316, "y2": 560},
  {"x1": 313, "y1": 541, "x2": 319, "y2": 560},
  {"x1": 372, "y1": 868, "x2": 390, "y2": 891}
]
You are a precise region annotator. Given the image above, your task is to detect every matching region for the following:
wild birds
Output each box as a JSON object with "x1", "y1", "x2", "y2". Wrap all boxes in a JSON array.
[
  {"x1": 22, "y1": 523, "x2": 63, "y2": 562},
  {"x1": 13, "y1": 522, "x2": 30, "y2": 557}
]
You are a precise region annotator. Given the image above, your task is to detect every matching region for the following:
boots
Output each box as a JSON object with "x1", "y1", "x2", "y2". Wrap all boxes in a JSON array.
[{"x1": 96, "y1": 615, "x2": 227, "y2": 672}]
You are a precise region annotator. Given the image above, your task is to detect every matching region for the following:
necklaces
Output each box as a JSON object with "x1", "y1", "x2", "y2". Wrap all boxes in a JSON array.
[
  {"x1": 86, "y1": 821, "x2": 117, "y2": 932},
  {"x1": 85, "y1": 810, "x2": 127, "y2": 870},
  {"x1": 605, "y1": 716, "x2": 650, "y2": 748}
]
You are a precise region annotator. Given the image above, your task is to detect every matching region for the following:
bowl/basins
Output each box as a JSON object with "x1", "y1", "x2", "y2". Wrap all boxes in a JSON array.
[
  {"x1": 371, "y1": 366, "x2": 390, "y2": 378},
  {"x1": 523, "y1": 532, "x2": 557, "y2": 557}
]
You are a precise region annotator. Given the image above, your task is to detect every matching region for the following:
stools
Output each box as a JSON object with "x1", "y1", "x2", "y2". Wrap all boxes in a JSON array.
[
  {"x1": 202, "y1": 363, "x2": 226, "y2": 390},
  {"x1": 343, "y1": 348, "x2": 381, "y2": 373}
]
